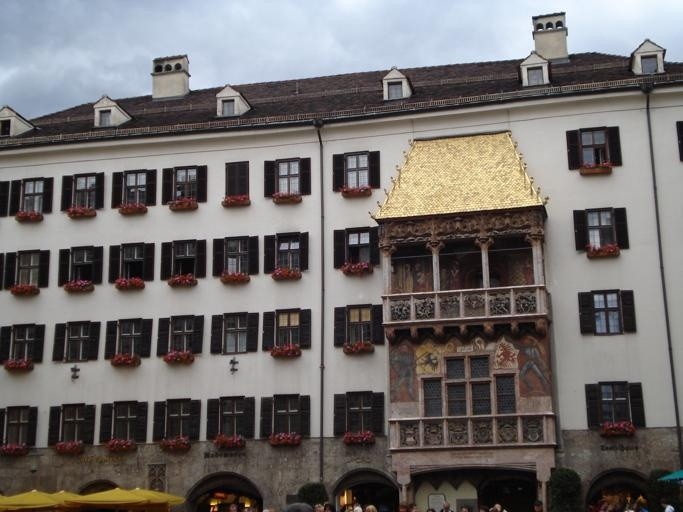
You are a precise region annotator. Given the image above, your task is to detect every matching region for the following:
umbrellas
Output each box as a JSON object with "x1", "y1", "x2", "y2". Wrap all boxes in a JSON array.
[{"x1": 0, "y1": 487, "x2": 185, "y2": 511}]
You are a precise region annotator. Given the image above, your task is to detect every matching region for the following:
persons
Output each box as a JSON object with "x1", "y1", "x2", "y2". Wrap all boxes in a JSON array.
[
  {"x1": 209, "y1": 491, "x2": 683, "y2": 512},
  {"x1": 505, "y1": 334, "x2": 553, "y2": 395},
  {"x1": 391, "y1": 344, "x2": 418, "y2": 402}
]
